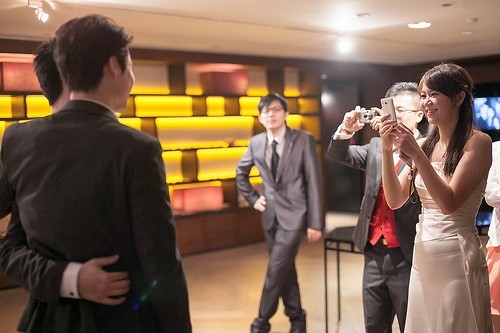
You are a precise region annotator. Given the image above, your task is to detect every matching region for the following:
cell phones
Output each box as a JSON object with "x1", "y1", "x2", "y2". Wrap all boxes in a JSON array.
[{"x1": 380, "y1": 98, "x2": 396, "y2": 123}]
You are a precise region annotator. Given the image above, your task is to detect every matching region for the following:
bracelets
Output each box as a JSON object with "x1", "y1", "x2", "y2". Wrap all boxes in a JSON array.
[{"x1": 343, "y1": 128, "x2": 353, "y2": 134}]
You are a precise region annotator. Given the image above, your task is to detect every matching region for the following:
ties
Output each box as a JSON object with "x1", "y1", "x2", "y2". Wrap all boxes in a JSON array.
[{"x1": 271, "y1": 140, "x2": 279, "y2": 182}]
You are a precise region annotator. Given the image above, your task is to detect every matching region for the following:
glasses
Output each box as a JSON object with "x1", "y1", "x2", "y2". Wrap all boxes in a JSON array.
[
  {"x1": 397, "y1": 107, "x2": 425, "y2": 117},
  {"x1": 261, "y1": 107, "x2": 283, "y2": 114}
]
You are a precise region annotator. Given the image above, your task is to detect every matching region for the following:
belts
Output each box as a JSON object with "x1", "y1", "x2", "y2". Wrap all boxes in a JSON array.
[{"x1": 375, "y1": 238, "x2": 388, "y2": 246}]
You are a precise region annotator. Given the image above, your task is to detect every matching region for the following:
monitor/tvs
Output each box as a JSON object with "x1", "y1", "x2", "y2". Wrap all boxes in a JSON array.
[{"x1": 472, "y1": 96, "x2": 500, "y2": 132}]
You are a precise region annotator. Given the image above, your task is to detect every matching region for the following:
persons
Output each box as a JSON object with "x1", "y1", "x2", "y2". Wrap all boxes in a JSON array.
[
  {"x1": 379, "y1": 62, "x2": 493, "y2": 333},
  {"x1": 328, "y1": 82, "x2": 425, "y2": 333},
  {"x1": 483, "y1": 140, "x2": 500, "y2": 333},
  {"x1": 0, "y1": 14, "x2": 193, "y2": 333},
  {"x1": 236, "y1": 92, "x2": 326, "y2": 333},
  {"x1": 0, "y1": 36, "x2": 130, "y2": 304}
]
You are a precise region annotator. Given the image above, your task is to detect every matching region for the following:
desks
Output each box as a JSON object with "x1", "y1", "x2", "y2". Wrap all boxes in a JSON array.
[{"x1": 324, "y1": 226, "x2": 364, "y2": 333}]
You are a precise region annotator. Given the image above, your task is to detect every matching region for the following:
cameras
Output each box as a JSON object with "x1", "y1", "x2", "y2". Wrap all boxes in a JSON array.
[{"x1": 358, "y1": 109, "x2": 377, "y2": 123}]
